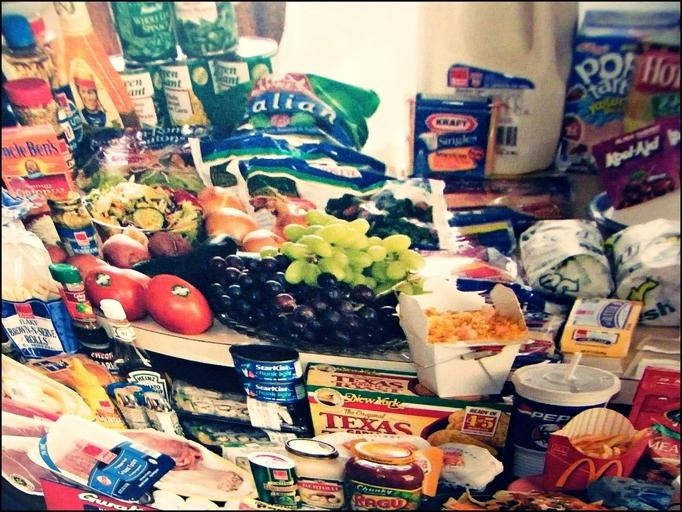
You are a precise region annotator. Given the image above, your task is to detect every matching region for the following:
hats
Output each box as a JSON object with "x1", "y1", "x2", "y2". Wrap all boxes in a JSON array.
[{"x1": 71, "y1": 68, "x2": 100, "y2": 90}]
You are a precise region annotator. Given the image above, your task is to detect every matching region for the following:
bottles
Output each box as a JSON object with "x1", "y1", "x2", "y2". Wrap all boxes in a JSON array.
[
  {"x1": 49, "y1": 265, "x2": 128, "y2": 383},
  {"x1": 2, "y1": 14, "x2": 78, "y2": 185},
  {"x1": 99, "y1": 298, "x2": 174, "y2": 403}
]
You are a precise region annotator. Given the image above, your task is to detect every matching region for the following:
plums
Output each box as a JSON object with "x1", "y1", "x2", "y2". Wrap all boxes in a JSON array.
[{"x1": 149, "y1": 232, "x2": 192, "y2": 258}]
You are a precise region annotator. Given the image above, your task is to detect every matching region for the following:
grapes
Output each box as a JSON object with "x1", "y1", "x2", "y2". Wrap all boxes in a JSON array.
[{"x1": 204, "y1": 210, "x2": 428, "y2": 349}]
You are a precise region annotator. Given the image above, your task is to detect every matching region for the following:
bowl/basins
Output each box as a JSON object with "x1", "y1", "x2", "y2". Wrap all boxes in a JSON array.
[
  {"x1": 585, "y1": 190, "x2": 629, "y2": 237},
  {"x1": 82, "y1": 190, "x2": 206, "y2": 243}
]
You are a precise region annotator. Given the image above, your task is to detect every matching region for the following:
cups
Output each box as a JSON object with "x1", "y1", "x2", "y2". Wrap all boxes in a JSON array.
[
  {"x1": 511, "y1": 362, "x2": 621, "y2": 478},
  {"x1": 227, "y1": 344, "x2": 317, "y2": 437}
]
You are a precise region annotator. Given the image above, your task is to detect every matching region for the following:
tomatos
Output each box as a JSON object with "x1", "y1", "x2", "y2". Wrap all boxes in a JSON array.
[{"x1": 46, "y1": 243, "x2": 213, "y2": 336}]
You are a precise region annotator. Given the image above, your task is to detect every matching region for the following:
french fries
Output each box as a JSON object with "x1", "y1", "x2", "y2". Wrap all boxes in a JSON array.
[{"x1": 570, "y1": 426, "x2": 651, "y2": 460}]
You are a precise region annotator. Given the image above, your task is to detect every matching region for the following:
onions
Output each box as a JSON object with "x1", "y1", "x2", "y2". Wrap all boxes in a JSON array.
[{"x1": 198, "y1": 185, "x2": 315, "y2": 253}]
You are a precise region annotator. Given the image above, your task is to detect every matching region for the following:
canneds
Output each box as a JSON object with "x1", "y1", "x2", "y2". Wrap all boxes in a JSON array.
[
  {"x1": 229, "y1": 345, "x2": 307, "y2": 405},
  {"x1": 248, "y1": 437, "x2": 425, "y2": 512},
  {"x1": 110, "y1": 2, "x2": 273, "y2": 133}
]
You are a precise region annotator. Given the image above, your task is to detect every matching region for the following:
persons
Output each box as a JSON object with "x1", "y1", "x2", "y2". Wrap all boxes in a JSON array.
[{"x1": 71, "y1": 70, "x2": 107, "y2": 128}]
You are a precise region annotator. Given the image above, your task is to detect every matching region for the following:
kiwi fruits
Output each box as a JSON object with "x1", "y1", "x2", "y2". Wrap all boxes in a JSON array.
[{"x1": 102, "y1": 233, "x2": 147, "y2": 268}]
